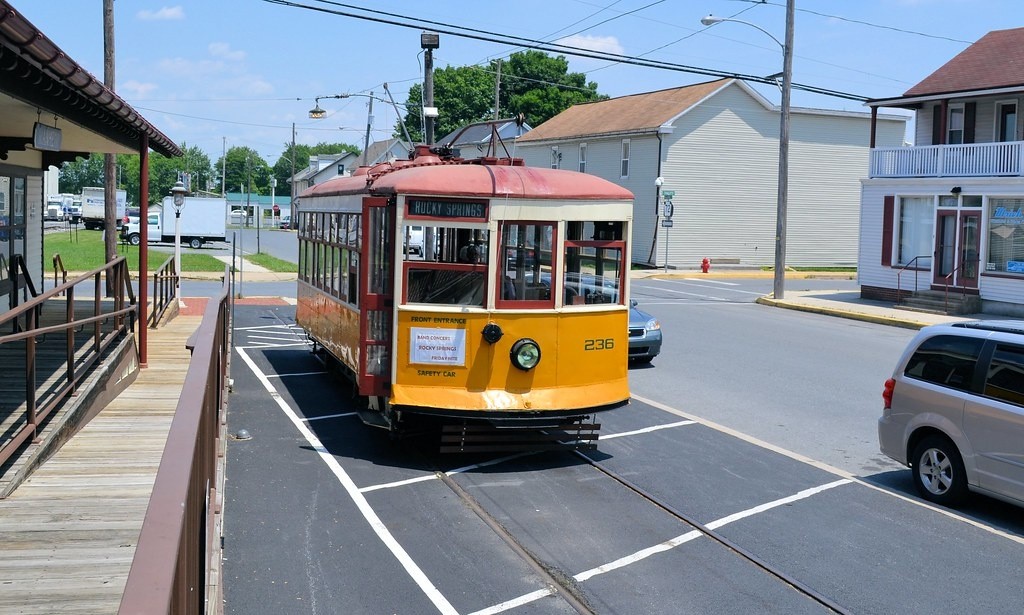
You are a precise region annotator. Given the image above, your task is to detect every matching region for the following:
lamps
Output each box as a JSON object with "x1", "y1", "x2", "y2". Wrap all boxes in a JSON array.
[{"x1": 950, "y1": 187, "x2": 962, "y2": 199}]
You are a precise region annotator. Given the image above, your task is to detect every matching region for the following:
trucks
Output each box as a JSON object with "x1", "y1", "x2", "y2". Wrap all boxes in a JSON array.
[
  {"x1": 120, "y1": 197, "x2": 232, "y2": 248},
  {"x1": 82, "y1": 187, "x2": 129, "y2": 230},
  {"x1": 44, "y1": 193, "x2": 82, "y2": 224}
]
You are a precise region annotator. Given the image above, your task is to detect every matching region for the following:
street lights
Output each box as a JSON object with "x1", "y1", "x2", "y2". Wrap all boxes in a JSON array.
[
  {"x1": 267, "y1": 153, "x2": 294, "y2": 229},
  {"x1": 171, "y1": 180, "x2": 187, "y2": 305},
  {"x1": 246, "y1": 165, "x2": 264, "y2": 227},
  {"x1": 702, "y1": 16, "x2": 792, "y2": 300},
  {"x1": 340, "y1": 126, "x2": 369, "y2": 165}
]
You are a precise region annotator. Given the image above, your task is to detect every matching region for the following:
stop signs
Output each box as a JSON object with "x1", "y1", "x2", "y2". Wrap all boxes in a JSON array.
[{"x1": 273, "y1": 205, "x2": 279, "y2": 212}]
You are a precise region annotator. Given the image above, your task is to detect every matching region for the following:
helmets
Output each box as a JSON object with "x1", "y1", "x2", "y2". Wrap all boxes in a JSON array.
[
  {"x1": 466, "y1": 245, "x2": 481, "y2": 259},
  {"x1": 460, "y1": 247, "x2": 468, "y2": 258}
]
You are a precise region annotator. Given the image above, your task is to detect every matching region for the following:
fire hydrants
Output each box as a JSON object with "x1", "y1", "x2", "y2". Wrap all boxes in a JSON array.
[{"x1": 701, "y1": 257, "x2": 710, "y2": 274}]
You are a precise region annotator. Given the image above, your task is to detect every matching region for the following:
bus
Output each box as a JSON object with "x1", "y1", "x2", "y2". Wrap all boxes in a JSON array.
[{"x1": 294, "y1": 148, "x2": 635, "y2": 452}]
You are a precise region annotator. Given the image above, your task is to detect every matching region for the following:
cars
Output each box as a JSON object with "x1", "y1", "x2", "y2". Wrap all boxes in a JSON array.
[
  {"x1": 419, "y1": 234, "x2": 448, "y2": 257},
  {"x1": 522, "y1": 272, "x2": 661, "y2": 365},
  {"x1": 280, "y1": 216, "x2": 292, "y2": 228},
  {"x1": 477, "y1": 240, "x2": 534, "y2": 268}
]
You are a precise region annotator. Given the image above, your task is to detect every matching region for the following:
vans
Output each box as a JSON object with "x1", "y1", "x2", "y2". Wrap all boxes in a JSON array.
[
  {"x1": 403, "y1": 226, "x2": 424, "y2": 252},
  {"x1": 878, "y1": 317, "x2": 1024, "y2": 511}
]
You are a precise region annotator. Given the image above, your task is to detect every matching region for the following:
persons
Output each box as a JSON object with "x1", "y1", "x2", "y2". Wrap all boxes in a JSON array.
[
  {"x1": 417, "y1": 245, "x2": 484, "y2": 305},
  {"x1": 505, "y1": 276, "x2": 516, "y2": 299}
]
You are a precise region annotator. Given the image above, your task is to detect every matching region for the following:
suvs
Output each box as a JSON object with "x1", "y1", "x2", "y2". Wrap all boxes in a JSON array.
[{"x1": 231, "y1": 210, "x2": 248, "y2": 219}]
